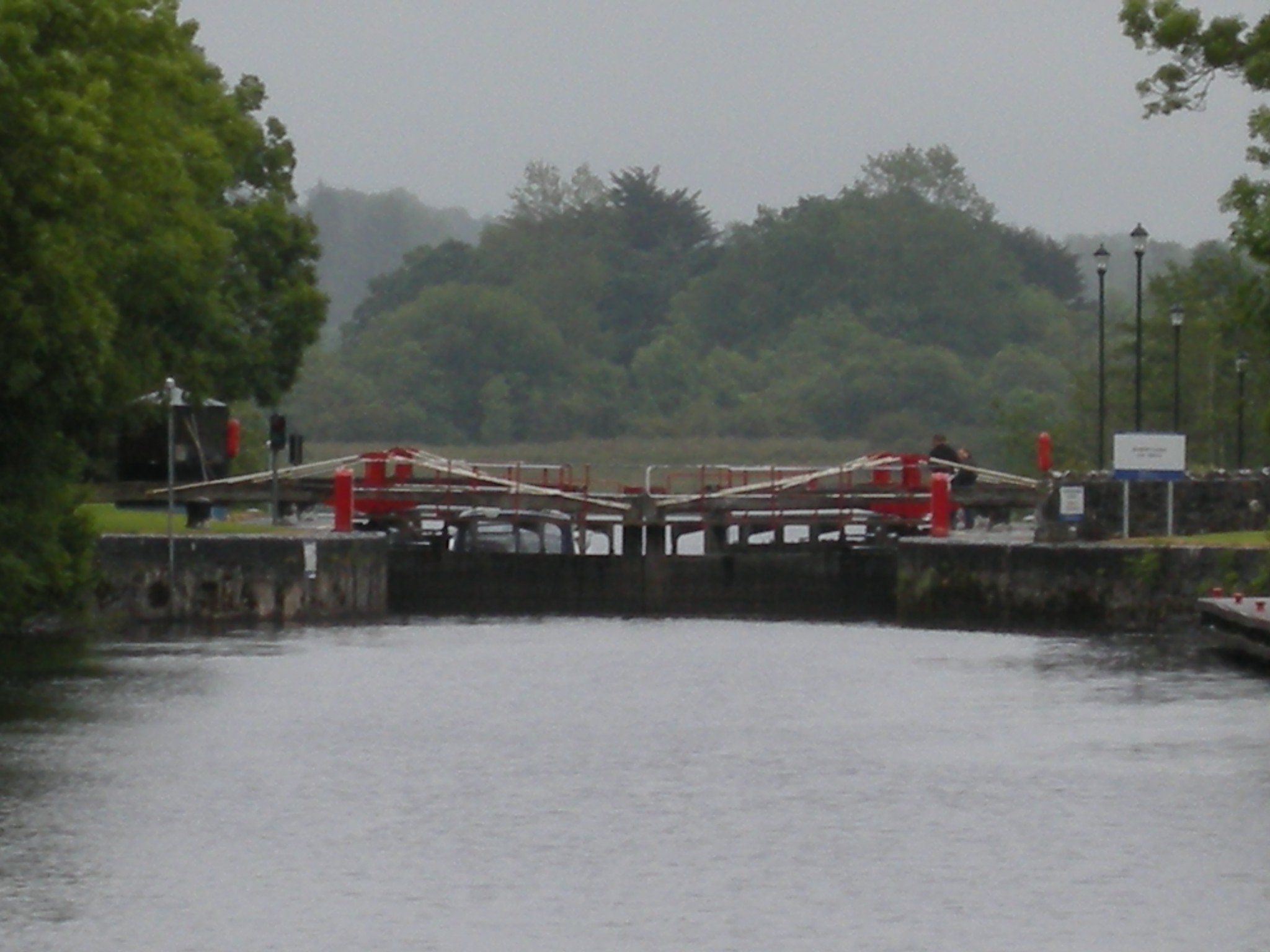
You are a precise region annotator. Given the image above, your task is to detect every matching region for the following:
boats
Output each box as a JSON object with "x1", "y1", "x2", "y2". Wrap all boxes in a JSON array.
[{"x1": 1196, "y1": 585, "x2": 1270, "y2": 664}]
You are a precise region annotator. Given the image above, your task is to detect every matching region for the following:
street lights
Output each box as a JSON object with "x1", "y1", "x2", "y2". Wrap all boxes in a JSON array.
[
  {"x1": 1170, "y1": 304, "x2": 1186, "y2": 439},
  {"x1": 1128, "y1": 222, "x2": 1151, "y2": 432},
  {"x1": 1234, "y1": 352, "x2": 1251, "y2": 477},
  {"x1": 1093, "y1": 242, "x2": 1111, "y2": 470}
]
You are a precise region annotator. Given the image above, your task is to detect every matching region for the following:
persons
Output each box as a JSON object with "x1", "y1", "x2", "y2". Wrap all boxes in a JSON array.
[
  {"x1": 929, "y1": 434, "x2": 958, "y2": 491},
  {"x1": 949, "y1": 447, "x2": 980, "y2": 531}
]
